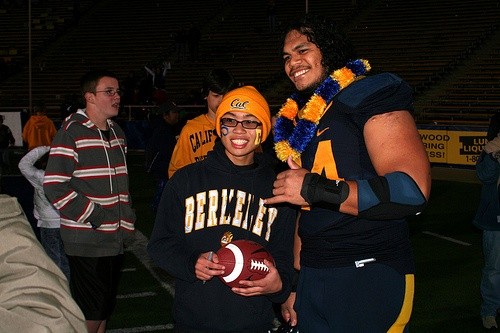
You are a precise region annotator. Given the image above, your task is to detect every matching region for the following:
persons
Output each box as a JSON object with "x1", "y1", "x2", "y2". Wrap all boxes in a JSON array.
[
  {"x1": 148, "y1": 102, "x2": 181, "y2": 213},
  {"x1": 43, "y1": 72, "x2": 136, "y2": 333},
  {"x1": 169, "y1": 69, "x2": 237, "y2": 178},
  {"x1": 268, "y1": 0, "x2": 276, "y2": 29},
  {"x1": 264, "y1": 15, "x2": 432, "y2": 333},
  {"x1": 23, "y1": 104, "x2": 57, "y2": 169},
  {"x1": 0, "y1": 115, "x2": 15, "y2": 148},
  {"x1": 475, "y1": 112, "x2": 500, "y2": 333},
  {"x1": 147, "y1": 86, "x2": 300, "y2": 333},
  {"x1": 0, "y1": 194, "x2": 90, "y2": 333},
  {"x1": 176, "y1": 23, "x2": 201, "y2": 64},
  {"x1": 19, "y1": 146, "x2": 71, "y2": 280}
]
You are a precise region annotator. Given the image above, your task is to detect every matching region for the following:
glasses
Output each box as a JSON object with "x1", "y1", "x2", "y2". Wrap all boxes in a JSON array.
[
  {"x1": 222, "y1": 117, "x2": 262, "y2": 130},
  {"x1": 90, "y1": 88, "x2": 120, "y2": 97}
]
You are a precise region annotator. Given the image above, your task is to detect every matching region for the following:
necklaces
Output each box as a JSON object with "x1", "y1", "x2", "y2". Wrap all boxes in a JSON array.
[{"x1": 254, "y1": 47, "x2": 371, "y2": 162}]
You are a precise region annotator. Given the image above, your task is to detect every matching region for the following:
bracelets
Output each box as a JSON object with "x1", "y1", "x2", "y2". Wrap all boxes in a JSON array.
[
  {"x1": 300, "y1": 174, "x2": 350, "y2": 213},
  {"x1": 290, "y1": 268, "x2": 300, "y2": 293}
]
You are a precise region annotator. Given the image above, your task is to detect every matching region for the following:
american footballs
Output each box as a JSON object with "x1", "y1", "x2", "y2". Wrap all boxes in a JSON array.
[{"x1": 213, "y1": 240, "x2": 278, "y2": 289}]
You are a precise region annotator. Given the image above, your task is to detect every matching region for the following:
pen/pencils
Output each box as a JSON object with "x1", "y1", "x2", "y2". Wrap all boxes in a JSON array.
[{"x1": 203, "y1": 252, "x2": 214, "y2": 285}]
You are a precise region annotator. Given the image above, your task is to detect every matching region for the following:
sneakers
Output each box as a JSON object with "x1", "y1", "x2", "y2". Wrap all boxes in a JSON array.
[{"x1": 483, "y1": 316, "x2": 497, "y2": 330}]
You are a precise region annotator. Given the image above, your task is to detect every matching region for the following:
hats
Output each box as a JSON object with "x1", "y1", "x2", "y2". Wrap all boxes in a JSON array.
[
  {"x1": 158, "y1": 99, "x2": 180, "y2": 113},
  {"x1": 215, "y1": 86, "x2": 270, "y2": 144}
]
